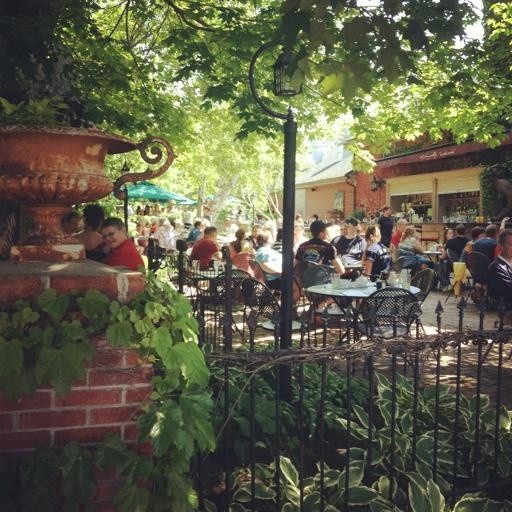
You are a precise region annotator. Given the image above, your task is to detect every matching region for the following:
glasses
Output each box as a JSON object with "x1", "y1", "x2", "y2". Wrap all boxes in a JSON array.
[{"x1": 102, "y1": 231, "x2": 114, "y2": 238}]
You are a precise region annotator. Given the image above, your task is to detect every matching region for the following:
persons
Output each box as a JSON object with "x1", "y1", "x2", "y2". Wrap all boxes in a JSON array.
[
  {"x1": 72, "y1": 204, "x2": 106, "y2": 264},
  {"x1": 53, "y1": 213, "x2": 82, "y2": 242},
  {"x1": 102, "y1": 217, "x2": 145, "y2": 274},
  {"x1": 139, "y1": 207, "x2": 510, "y2": 326}
]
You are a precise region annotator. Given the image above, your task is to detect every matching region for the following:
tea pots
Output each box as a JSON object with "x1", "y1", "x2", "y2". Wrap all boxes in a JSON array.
[
  {"x1": 357, "y1": 274, "x2": 368, "y2": 286},
  {"x1": 335, "y1": 279, "x2": 352, "y2": 289}
]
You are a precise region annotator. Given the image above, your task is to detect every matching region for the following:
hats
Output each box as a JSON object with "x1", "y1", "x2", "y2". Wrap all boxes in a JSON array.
[{"x1": 310, "y1": 221, "x2": 330, "y2": 230}]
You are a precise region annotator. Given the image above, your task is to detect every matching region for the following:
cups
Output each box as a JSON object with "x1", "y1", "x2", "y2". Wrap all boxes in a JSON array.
[
  {"x1": 376, "y1": 280, "x2": 386, "y2": 291},
  {"x1": 430, "y1": 243, "x2": 444, "y2": 252},
  {"x1": 330, "y1": 273, "x2": 340, "y2": 289},
  {"x1": 380, "y1": 271, "x2": 389, "y2": 286},
  {"x1": 389, "y1": 269, "x2": 412, "y2": 291}
]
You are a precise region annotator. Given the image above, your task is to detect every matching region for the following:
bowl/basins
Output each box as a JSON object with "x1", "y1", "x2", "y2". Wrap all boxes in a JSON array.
[{"x1": 347, "y1": 260, "x2": 358, "y2": 265}]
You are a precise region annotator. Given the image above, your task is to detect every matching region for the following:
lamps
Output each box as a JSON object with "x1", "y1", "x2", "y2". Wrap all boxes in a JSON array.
[{"x1": 369, "y1": 175, "x2": 386, "y2": 193}]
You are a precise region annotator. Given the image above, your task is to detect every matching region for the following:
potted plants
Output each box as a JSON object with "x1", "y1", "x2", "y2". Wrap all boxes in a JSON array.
[
  {"x1": 0, "y1": 93, "x2": 140, "y2": 264},
  {"x1": 131, "y1": 213, "x2": 157, "y2": 247}
]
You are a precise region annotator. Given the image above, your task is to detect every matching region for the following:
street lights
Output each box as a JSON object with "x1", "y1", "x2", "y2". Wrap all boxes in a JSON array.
[{"x1": 249, "y1": 38, "x2": 305, "y2": 356}]
